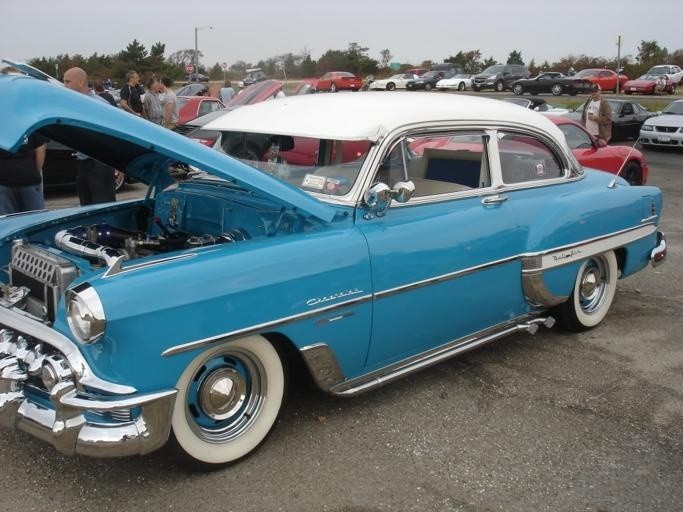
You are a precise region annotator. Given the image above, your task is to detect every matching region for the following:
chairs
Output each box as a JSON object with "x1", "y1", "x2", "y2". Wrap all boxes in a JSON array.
[{"x1": 414, "y1": 143, "x2": 486, "y2": 189}]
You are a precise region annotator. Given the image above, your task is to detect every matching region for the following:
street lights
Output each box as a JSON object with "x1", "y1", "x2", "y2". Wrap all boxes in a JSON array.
[{"x1": 194, "y1": 23, "x2": 214, "y2": 83}]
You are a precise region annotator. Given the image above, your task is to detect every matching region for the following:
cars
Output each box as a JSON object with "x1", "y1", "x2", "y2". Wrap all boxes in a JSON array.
[
  {"x1": 1, "y1": 57, "x2": 373, "y2": 167},
  {"x1": 406, "y1": 112, "x2": 649, "y2": 187},
  {"x1": 436, "y1": 74, "x2": 477, "y2": 91},
  {"x1": 639, "y1": 65, "x2": 683, "y2": 86},
  {"x1": 316, "y1": 71, "x2": 363, "y2": 93},
  {"x1": 561, "y1": 98, "x2": 658, "y2": 143},
  {"x1": 404, "y1": 68, "x2": 428, "y2": 76},
  {"x1": 0, "y1": 54, "x2": 665, "y2": 471},
  {"x1": 510, "y1": 71, "x2": 593, "y2": 96},
  {"x1": 571, "y1": 68, "x2": 628, "y2": 93},
  {"x1": 405, "y1": 70, "x2": 455, "y2": 92},
  {"x1": 369, "y1": 73, "x2": 419, "y2": 91},
  {"x1": 639, "y1": 99, "x2": 683, "y2": 150}
]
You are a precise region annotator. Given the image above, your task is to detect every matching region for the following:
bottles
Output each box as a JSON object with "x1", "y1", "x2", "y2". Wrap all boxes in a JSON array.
[{"x1": 587, "y1": 105, "x2": 593, "y2": 120}]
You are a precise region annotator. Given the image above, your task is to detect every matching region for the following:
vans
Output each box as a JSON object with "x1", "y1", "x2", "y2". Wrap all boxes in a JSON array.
[{"x1": 430, "y1": 63, "x2": 464, "y2": 76}]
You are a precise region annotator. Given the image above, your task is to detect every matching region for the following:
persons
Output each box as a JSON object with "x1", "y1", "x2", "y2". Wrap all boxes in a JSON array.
[
  {"x1": 91, "y1": 70, "x2": 179, "y2": 133},
  {"x1": 201, "y1": 77, "x2": 294, "y2": 174},
  {"x1": 361, "y1": 74, "x2": 375, "y2": 90},
  {"x1": 580, "y1": 82, "x2": 612, "y2": 144},
  {"x1": 527, "y1": 70, "x2": 532, "y2": 78},
  {"x1": 616, "y1": 66, "x2": 624, "y2": 75},
  {"x1": 436, "y1": 73, "x2": 442, "y2": 80},
  {"x1": 654, "y1": 75, "x2": 666, "y2": 96},
  {"x1": 567, "y1": 65, "x2": 576, "y2": 77},
  {"x1": 539, "y1": 70, "x2": 543, "y2": 74},
  {"x1": 1, "y1": 65, "x2": 47, "y2": 216},
  {"x1": 62, "y1": 67, "x2": 123, "y2": 205}
]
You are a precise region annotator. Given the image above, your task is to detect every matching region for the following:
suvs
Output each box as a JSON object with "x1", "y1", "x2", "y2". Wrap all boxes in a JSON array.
[{"x1": 470, "y1": 64, "x2": 533, "y2": 92}]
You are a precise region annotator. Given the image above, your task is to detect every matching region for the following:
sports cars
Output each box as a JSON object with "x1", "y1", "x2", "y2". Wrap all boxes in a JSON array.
[{"x1": 624, "y1": 74, "x2": 677, "y2": 95}]
